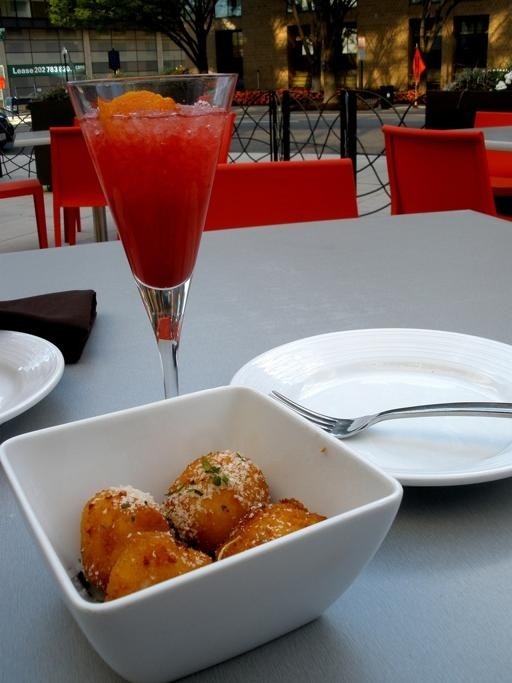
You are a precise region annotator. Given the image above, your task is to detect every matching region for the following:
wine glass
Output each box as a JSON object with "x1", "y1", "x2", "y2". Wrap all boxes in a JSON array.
[{"x1": 66, "y1": 73, "x2": 239, "y2": 400}]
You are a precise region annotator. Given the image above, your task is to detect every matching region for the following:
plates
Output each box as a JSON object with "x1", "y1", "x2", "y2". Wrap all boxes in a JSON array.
[
  {"x1": 0, "y1": 328, "x2": 66, "y2": 426},
  {"x1": 230, "y1": 327, "x2": 512, "y2": 488}
]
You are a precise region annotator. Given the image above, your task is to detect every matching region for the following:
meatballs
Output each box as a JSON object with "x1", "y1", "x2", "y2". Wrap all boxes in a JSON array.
[{"x1": 79, "y1": 451, "x2": 327, "y2": 600}]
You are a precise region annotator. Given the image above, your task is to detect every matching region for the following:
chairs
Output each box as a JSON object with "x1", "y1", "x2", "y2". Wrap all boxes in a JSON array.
[
  {"x1": 382, "y1": 124, "x2": 498, "y2": 217},
  {"x1": 203, "y1": 158, "x2": 358, "y2": 231},
  {"x1": 0, "y1": 179, "x2": 49, "y2": 249},
  {"x1": 49, "y1": 127, "x2": 120, "y2": 248},
  {"x1": 475, "y1": 112, "x2": 512, "y2": 195}
]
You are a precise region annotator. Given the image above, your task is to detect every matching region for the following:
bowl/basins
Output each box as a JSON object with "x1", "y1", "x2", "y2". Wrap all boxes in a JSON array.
[{"x1": 0, "y1": 385, "x2": 405, "y2": 683}]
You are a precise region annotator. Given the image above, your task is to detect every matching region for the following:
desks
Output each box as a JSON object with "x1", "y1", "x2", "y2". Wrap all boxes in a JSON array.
[
  {"x1": 0, "y1": 209, "x2": 512, "y2": 683},
  {"x1": 0, "y1": 131, "x2": 109, "y2": 242},
  {"x1": 452, "y1": 125, "x2": 512, "y2": 152}
]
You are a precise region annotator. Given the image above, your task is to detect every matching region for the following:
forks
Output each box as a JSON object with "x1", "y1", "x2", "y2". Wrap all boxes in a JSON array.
[{"x1": 267, "y1": 389, "x2": 512, "y2": 441}]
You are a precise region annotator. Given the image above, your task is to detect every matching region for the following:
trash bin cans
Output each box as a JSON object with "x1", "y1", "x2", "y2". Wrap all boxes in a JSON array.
[
  {"x1": 425, "y1": 91, "x2": 464, "y2": 129},
  {"x1": 379, "y1": 85, "x2": 394, "y2": 110}
]
